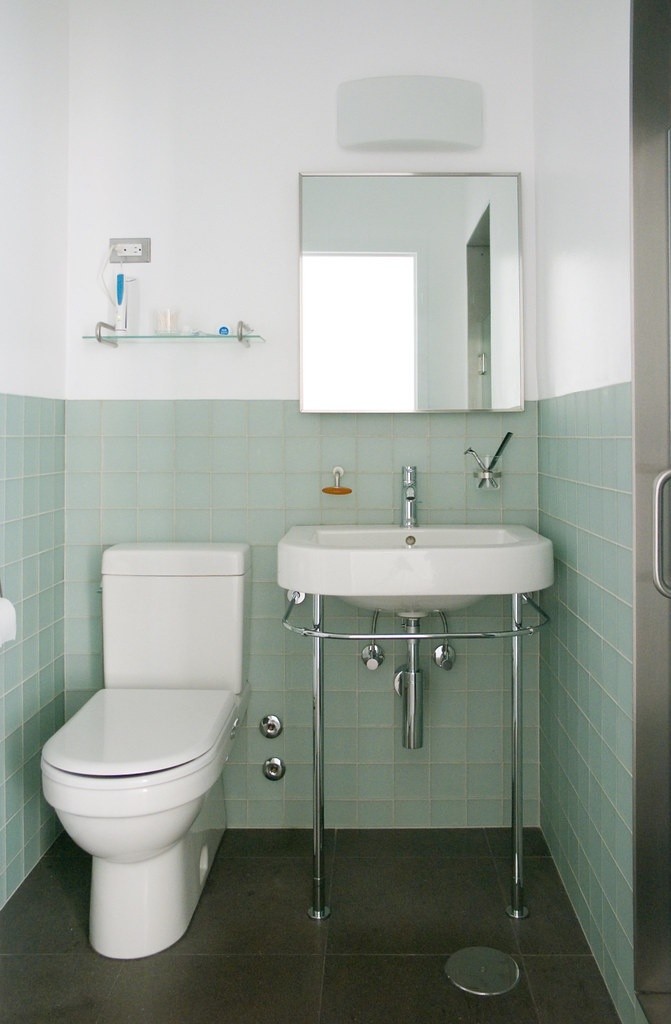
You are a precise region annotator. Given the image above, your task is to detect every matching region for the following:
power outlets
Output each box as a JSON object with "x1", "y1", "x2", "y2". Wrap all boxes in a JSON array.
[{"x1": 109, "y1": 237, "x2": 152, "y2": 264}]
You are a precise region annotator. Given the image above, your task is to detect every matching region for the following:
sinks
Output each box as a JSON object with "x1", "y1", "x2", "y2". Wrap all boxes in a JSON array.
[{"x1": 276, "y1": 526, "x2": 554, "y2": 619}]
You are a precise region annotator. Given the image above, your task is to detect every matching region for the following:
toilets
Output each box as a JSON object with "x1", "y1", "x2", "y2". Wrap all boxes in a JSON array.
[{"x1": 38, "y1": 540, "x2": 254, "y2": 962}]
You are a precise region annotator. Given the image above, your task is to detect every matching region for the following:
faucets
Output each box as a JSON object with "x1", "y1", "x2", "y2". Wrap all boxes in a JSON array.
[{"x1": 400, "y1": 465, "x2": 417, "y2": 526}]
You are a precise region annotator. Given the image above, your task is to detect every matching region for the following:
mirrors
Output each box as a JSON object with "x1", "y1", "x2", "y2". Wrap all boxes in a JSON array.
[{"x1": 298, "y1": 171, "x2": 525, "y2": 415}]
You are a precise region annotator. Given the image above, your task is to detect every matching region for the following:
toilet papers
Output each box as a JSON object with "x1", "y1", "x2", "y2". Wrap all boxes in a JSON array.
[{"x1": 0, "y1": 597, "x2": 17, "y2": 648}]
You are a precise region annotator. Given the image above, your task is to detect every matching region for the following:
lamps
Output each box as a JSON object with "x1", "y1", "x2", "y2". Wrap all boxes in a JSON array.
[{"x1": 337, "y1": 75, "x2": 481, "y2": 152}]
